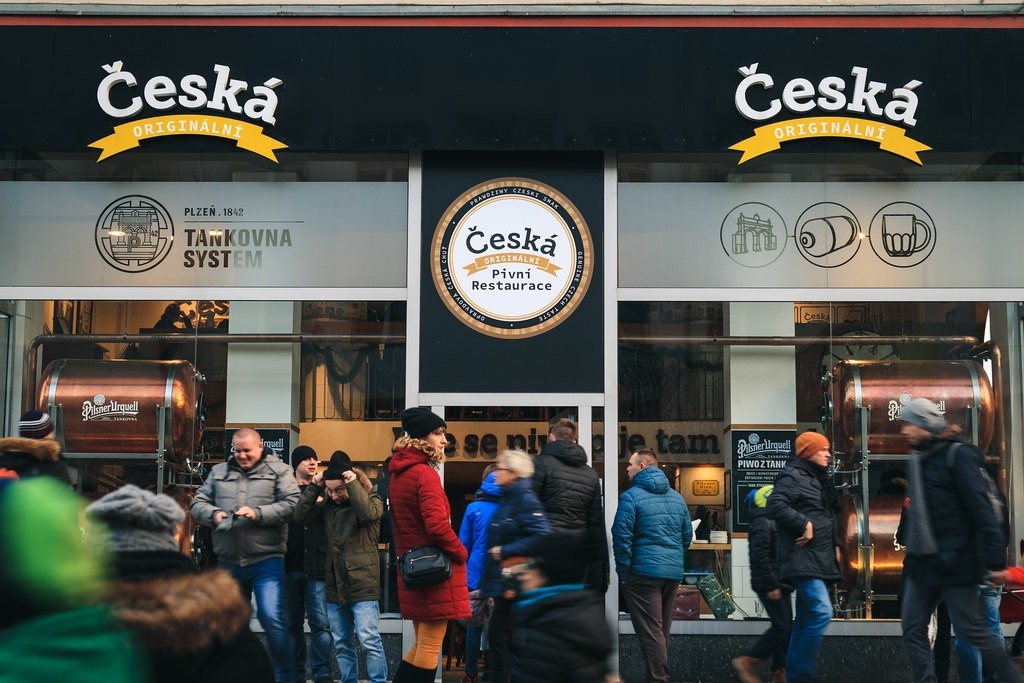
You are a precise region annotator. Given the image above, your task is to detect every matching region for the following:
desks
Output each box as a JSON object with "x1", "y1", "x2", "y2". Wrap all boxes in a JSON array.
[{"x1": 688, "y1": 543, "x2": 731, "y2": 588}]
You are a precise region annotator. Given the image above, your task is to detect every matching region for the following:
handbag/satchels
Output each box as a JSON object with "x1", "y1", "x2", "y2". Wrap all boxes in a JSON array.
[
  {"x1": 697, "y1": 574, "x2": 736, "y2": 621},
  {"x1": 399, "y1": 545, "x2": 452, "y2": 589},
  {"x1": 671, "y1": 586, "x2": 700, "y2": 620}
]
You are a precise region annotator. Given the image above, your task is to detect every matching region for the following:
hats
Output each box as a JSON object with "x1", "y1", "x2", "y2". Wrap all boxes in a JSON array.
[
  {"x1": 744, "y1": 485, "x2": 775, "y2": 511},
  {"x1": 795, "y1": 432, "x2": 830, "y2": 459},
  {"x1": 323, "y1": 450, "x2": 352, "y2": 480},
  {"x1": 401, "y1": 407, "x2": 448, "y2": 439},
  {"x1": 292, "y1": 446, "x2": 317, "y2": 468},
  {"x1": 900, "y1": 398, "x2": 944, "y2": 432},
  {"x1": 81, "y1": 484, "x2": 185, "y2": 552},
  {"x1": 19, "y1": 411, "x2": 54, "y2": 439}
]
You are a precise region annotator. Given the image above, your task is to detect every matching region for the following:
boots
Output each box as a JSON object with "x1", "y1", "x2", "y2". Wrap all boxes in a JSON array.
[{"x1": 392, "y1": 660, "x2": 438, "y2": 683}]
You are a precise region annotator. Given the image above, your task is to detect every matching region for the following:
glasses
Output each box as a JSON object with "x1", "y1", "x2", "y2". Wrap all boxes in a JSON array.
[{"x1": 495, "y1": 467, "x2": 513, "y2": 472}]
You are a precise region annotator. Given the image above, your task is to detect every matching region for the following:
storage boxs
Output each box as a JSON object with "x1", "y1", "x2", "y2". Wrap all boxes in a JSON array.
[{"x1": 671, "y1": 572, "x2": 736, "y2": 621}]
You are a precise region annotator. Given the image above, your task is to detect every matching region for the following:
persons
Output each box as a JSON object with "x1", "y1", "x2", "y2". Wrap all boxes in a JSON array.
[
  {"x1": 611, "y1": 450, "x2": 693, "y2": 683},
  {"x1": 480, "y1": 450, "x2": 549, "y2": 683},
  {"x1": 85, "y1": 485, "x2": 276, "y2": 683},
  {"x1": 154, "y1": 303, "x2": 194, "y2": 329},
  {"x1": 0, "y1": 411, "x2": 71, "y2": 485},
  {"x1": 505, "y1": 533, "x2": 613, "y2": 683},
  {"x1": 458, "y1": 465, "x2": 501, "y2": 683},
  {"x1": 732, "y1": 486, "x2": 793, "y2": 683},
  {"x1": 285, "y1": 445, "x2": 334, "y2": 683},
  {"x1": 190, "y1": 427, "x2": 300, "y2": 683},
  {"x1": 766, "y1": 432, "x2": 844, "y2": 683},
  {"x1": 0, "y1": 477, "x2": 146, "y2": 683},
  {"x1": 532, "y1": 419, "x2": 610, "y2": 596},
  {"x1": 295, "y1": 450, "x2": 389, "y2": 683},
  {"x1": 389, "y1": 407, "x2": 472, "y2": 683},
  {"x1": 897, "y1": 398, "x2": 1024, "y2": 683}
]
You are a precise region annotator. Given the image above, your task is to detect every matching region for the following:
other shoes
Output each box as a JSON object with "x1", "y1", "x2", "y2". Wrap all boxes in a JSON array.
[
  {"x1": 732, "y1": 656, "x2": 763, "y2": 683},
  {"x1": 462, "y1": 672, "x2": 478, "y2": 683},
  {"x1": 771, "y1": 668, "x2": 786, "y2": 683}
]
